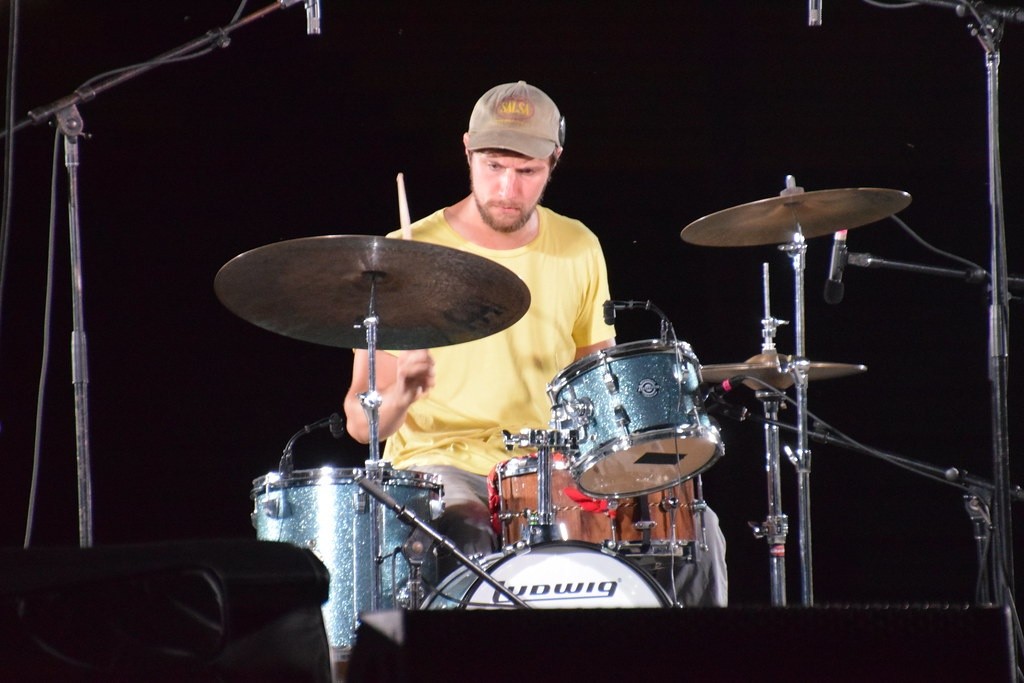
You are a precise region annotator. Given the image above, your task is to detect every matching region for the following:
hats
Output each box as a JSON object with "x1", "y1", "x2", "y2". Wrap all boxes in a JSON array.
[{"x1": 466, "y1": 81, "x2": 564, "y2": 158}]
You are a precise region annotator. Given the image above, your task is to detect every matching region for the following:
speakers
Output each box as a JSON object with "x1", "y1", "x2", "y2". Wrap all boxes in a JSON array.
[
  {"x1": 344, "y1": 607, "x2": 1016, "y2": 682},
  {"x1": 0, "y1": 541, "x2": 334, "y2": 683}
]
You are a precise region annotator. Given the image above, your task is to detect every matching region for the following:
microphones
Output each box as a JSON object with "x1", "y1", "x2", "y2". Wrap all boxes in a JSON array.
[
  {"x1": 702, "y1": 375, "x2": 746, "y2": 402},
  {"x1": 604, "y1": 300, "x2": 629, "y2": 326},
  {"x1": 320, "y1": 414, "x2": 343, "y2": 439},
  {"x1": 823, "y1": 230, "x2": 848, "y2": 303},
  {"x1": 305, "y1": 0, "x2": 321, "y2": 35},
  {"x1": 807, "y1": 0, "x2": 823, "y2": 26}
]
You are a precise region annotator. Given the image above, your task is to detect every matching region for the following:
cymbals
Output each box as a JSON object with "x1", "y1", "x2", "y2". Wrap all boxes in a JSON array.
[
  {"x1": 213, "y1": 235, "x2": 532, "y2": 351},
  {"x1": 698, "y1": 352, "x2": 867, "y2": 391},
  {"x1": 679, "y1": 187, "x2": 912, "y2": 248}
]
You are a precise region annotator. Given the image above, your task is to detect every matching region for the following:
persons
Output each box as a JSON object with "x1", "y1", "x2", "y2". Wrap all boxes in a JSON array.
[{"x1": 345, "y1": 81, "x2": 732, "y2": 610}]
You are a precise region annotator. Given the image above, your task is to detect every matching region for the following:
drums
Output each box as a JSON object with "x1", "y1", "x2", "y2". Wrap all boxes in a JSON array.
[
  {"x1": 543, "y1": 338, "x2": 725, "y2": 500},
  {"x1": 420, "y1": 538, "x2": 683, "y2": 608},
  {"x1": 249, "y1": 466, "x2": 447, "y2": 660},
  {"x1": 497, "y1": 458, "x2": 709, "y2": 571}
]
